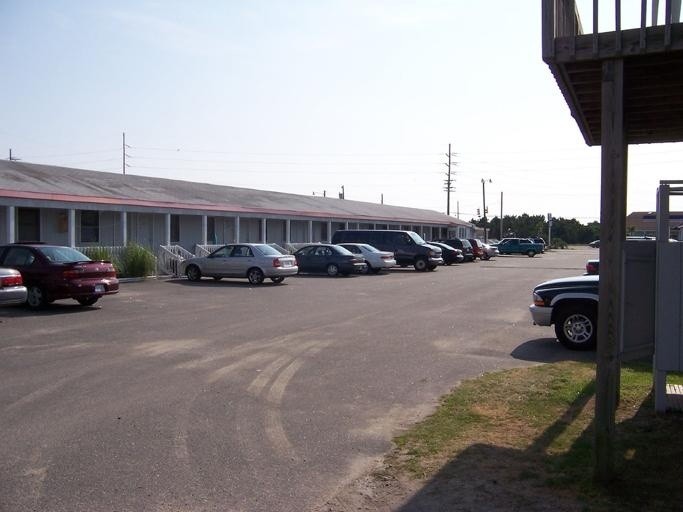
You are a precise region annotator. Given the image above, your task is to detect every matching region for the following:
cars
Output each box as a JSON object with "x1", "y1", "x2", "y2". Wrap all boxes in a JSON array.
[
  {"x1": 177, "y1": 242, "x2": 299, "y2": 284},
  {"x1": 427, "y1": 238, "x2": 500, "y2": 266},
  {"x1": 289, "y1": 242, "x2": 397, "y2": 278},
  {"x1": 526, "y1": 258, "x2": 599, "y2": 353},
  {"x1": 0, "y1": 241, "x2": 120, "y2": 310}
]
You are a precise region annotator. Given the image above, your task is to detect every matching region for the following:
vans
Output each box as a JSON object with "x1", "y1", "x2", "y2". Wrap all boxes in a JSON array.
[{"x1": 331, "y1": 229, "x2": 444, "y2": 273}]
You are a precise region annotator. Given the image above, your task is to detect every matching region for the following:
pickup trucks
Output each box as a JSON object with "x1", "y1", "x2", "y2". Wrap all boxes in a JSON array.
[{"x1": 489, "y1": 238, "x2": 545, "y2": 257}]
